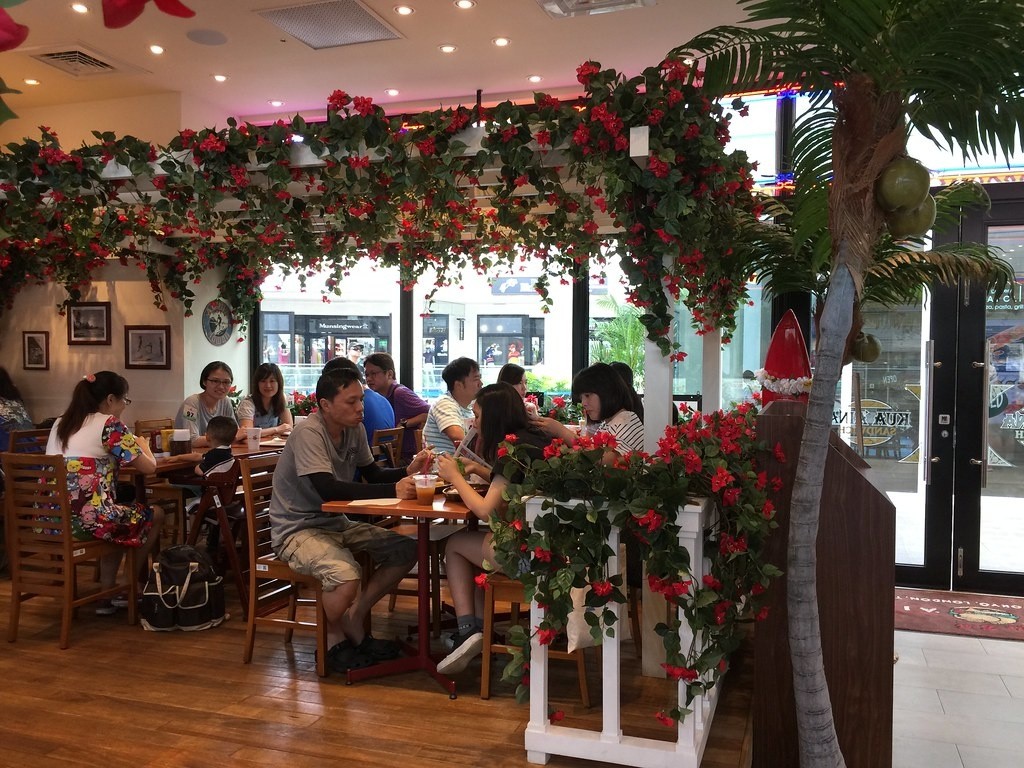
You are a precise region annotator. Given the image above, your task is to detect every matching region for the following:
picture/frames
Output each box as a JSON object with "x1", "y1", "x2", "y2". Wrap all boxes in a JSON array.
[
  {"x1": 22, "y1": 330, "x2": 50, "y2": 371},
  {"x1": 66, "y1": 301, "x2": 111, "y2": 345},
  {"x1": 124, "y1": 324, "x2": 171, "y2": 370}
]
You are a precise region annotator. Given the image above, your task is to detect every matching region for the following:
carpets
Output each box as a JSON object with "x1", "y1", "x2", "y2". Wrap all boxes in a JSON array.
[{"x1": 893, "y1": 588, "x2": 1024, "y2": 641}]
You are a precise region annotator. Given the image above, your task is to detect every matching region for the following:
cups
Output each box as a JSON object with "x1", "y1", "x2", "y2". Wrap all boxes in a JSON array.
[
  {"x1": 413, "y1": 475, "x2": 438, "y2": 505},
  {"x1": 152, "y1": 431, "x2": 191, "y2": 456},
  {"x1": 246, "y1": 428, "x2": 262, "y2": 449},
  {"x1": 579, "y1": 416, "x2": 586, "y2": 434}
]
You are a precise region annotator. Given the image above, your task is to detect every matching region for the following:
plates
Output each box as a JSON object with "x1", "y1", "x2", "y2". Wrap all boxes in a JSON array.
[
  {"x1": 442, "y1": 483, "x2": 490, "y2": 500},
  {"x1": 434, "y1": 480, "x2": 453, "y2": 493}
]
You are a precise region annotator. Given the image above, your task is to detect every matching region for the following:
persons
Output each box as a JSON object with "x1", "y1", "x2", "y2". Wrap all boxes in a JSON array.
[
  {"x1": 526, "y1": 362, "x2": 645, "y2": 464},
  {"x1": 322, "y1": 357, "x2": 395, "y2": 461},
  {"x1": 30, "y1": 371, "x2": 165, "y2": 614},
  {"x1": 743, "y1": 370, "x2": 760, "y2": 402},
  {"x1": 422, "y1": 346, "x2": 436, "y2": 384},
  {"x1": 348, "y1": 341, "x2": 364, "y2": 374},
  {"x1": 163, "y1": 416, "x2": 239, "y2": 545},
  {"x1": 436, "y1": 383, "x2": 553, "y2": 676},
  {"x1": 0, "y1": 367, "x2": 42, "y2": 486},
  {"x1": 611, "y1": 362, "x2": 644, "y2": 426},
  {"x1": 269, "y1": 369, "x2": 434, "y2": 674},
  {"x1": 236, "y1": 363, "x2": 293, "y2": 436},
  {"x1": 497, "y1": 363, "x2": 526, "y2": 399},
  {"x1": 364, "y1": 353, "x2": 432, "y2": 461},
  {"x1": 425, "y1": 358, "x2": 483, "y2": 455},
  {"x1": 174, "y1": 362, "x2": 249, "y2": 448}
]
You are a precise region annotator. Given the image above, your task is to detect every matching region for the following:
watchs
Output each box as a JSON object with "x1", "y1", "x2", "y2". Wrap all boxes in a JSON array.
[{"x1": 400, "y1": 418, "x2": 407, "y2": 428}]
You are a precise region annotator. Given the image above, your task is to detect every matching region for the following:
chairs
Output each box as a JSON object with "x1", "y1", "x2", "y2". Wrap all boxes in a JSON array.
[{"x1": 0, "y1": 416, "x2": 638, "y2": 712}]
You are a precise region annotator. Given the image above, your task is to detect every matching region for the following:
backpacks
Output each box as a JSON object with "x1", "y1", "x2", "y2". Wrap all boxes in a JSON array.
[{"x1": 142, "y1": 544, "x2": 225, "y2": 627}]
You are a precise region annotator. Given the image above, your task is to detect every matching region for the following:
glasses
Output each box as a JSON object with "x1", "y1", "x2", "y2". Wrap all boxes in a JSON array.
[
  {"x1": 105, "y1": 390, "x2": 131, "y2": 405},
  {"x1": 364, "y1": 368, "x2": 394, "y2": 378},
  {"x1": 206, "y1": 379, "x2": 232, "y2": 386}
]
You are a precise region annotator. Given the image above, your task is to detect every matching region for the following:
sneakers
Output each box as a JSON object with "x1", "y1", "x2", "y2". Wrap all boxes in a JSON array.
[
  {"x1": 315, "y1": 639, "x2": 375, "y2": 674},
  {"x1": 111, "y1": 591, "x2": 143, "y2": 607},
  {"x1": 437, "y1": 626, "x2": 483, "y2": 675},
  {"x1": 95, "y1": 599, "x2": 118, "y2": 614},
  {"x1": 351, "y1": 632, "x2": 400, "y2": 658},
  {"x1": 440, "y1": 631, "x2": 459, "y2": 649}
]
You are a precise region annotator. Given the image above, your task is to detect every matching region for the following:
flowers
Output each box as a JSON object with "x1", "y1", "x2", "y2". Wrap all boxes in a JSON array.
[
  {"x1": 288, "y1": 393, "x2": 319, "y2": 418},
  {"x1": 475, "y1": 430, "x2": 653, "y2": 722},
  {"x1": 0, "y1": 61, "x2": 787, "y2": 355},
  {"x1": 632, "y1": 389, "x2": 785, "y2": 726}
]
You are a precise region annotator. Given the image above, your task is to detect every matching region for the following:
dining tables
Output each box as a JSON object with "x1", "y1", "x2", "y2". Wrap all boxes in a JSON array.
[
  {"x1": 239, "y1": 431, "x2": 290, "y2": 449},
  {"x1": 113, "y1": 446, "x2": 281, "y2": 551},
  {"x1": 321, "y1": 482, "x2": 530, "y2": 700}
]
